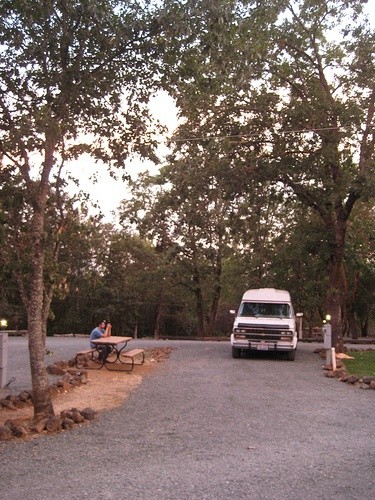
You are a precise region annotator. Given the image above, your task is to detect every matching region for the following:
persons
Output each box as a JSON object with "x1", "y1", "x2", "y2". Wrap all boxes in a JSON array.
[{"x1": 90, "y1": 320, "x2": 112, "y2": 362}]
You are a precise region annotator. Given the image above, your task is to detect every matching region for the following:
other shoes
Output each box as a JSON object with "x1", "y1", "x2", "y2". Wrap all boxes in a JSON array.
[{"x1": 98, "y1": 358, "x2": 107, "y2": 362}]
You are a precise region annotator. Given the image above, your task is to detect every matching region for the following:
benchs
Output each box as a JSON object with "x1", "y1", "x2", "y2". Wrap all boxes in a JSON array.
[
  {"x1": 121, "y1": 349, "x2": 145, "y2": 371},
  {"x1": 75, "y1": 349, "x2": 96, "y2": 370}
]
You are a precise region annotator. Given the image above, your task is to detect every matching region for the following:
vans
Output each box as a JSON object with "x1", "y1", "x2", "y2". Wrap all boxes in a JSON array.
[{"x1": 229, "y1": 288, "x2": 305, "y2": 361}]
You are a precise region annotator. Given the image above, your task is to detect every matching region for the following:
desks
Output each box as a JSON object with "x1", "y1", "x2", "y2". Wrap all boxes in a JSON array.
[{"x1": 90, "y1": 336, "x2": 132, "y2": 372}]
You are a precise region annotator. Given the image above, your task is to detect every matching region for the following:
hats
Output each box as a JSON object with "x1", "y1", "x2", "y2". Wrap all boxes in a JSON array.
[{"x1": 97, "y1": 319, "x2": 106, "y2": 323}]
